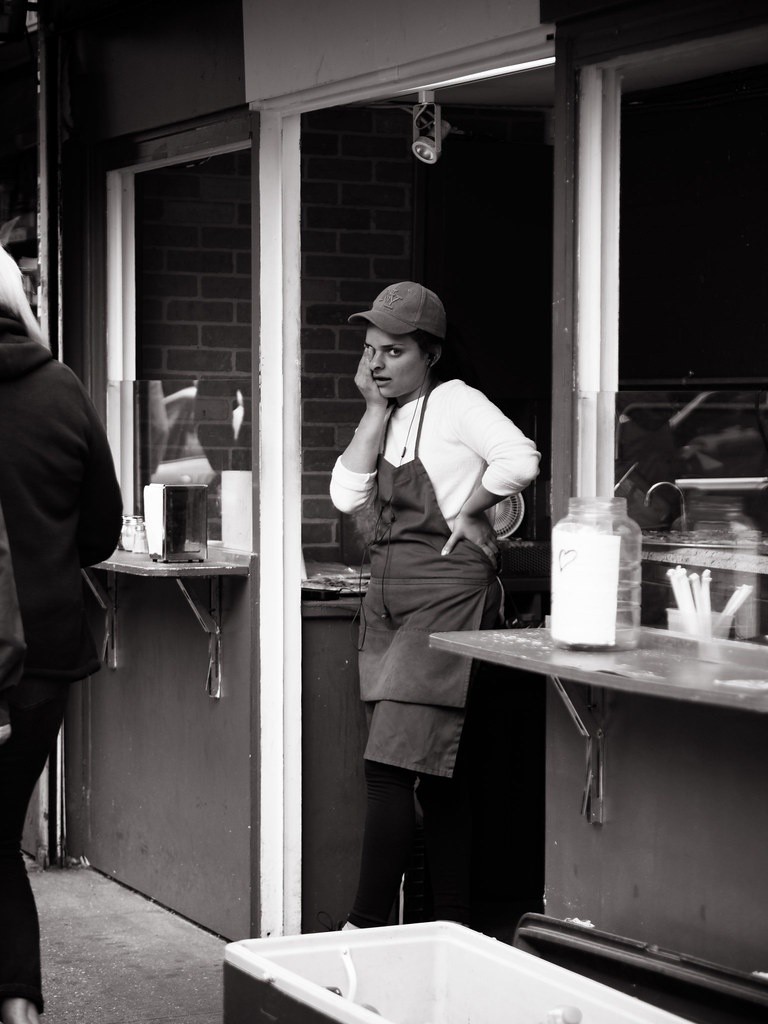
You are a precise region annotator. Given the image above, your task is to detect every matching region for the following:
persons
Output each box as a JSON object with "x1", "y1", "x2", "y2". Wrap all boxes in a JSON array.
[
  {"x1": 330, "y1": 278, "x2": 542, "y2": 930},
  {"x1": 0, "y1": 242, "x2": 123, "y2": 1024}
]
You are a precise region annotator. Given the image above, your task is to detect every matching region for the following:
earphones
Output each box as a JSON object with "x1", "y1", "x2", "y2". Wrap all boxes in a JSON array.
[
  {"x1": 382, "y1": 605, "x2": 389, "y2": 618},
  {"x1": 427, "y1": 352, "x2": 435, "y2": 369}
]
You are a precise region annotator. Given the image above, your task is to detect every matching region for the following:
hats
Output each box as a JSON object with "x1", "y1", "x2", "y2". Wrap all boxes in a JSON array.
[{"x1": 347, "y1": 281, "x2": 446, "y2": 338}]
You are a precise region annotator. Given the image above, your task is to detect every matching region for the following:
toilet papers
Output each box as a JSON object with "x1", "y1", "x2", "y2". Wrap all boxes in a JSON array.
[{"x1": 221, "y1": 468, "x2": 254, "y2": 548}]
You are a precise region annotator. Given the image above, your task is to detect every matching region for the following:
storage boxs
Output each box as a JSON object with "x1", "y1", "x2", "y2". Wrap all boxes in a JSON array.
[
  {"x1": 144, "y1": 483, "x2": 208, "y2": 563},
  {"x1": 223, "y1": 920, "x2": 694, "y2": 1024}
]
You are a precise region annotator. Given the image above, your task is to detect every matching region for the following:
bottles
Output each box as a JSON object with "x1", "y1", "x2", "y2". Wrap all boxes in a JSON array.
[
  {"x1": 549, "y1": 496, "x2": 645, "y2": 650},
  {"x1": 670, "y1": 497, "x2": 758, "y2": 639},
  {"x1": 133, "y1": 524, "x2": 147, "y2": 554}
]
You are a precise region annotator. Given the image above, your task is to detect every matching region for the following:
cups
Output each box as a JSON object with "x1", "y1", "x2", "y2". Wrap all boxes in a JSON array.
[
  {"x1": 121, "y1": 515, "x2": 143, "y2": 552},
  {"x1": 666, "y1": 608, "x2": 735, "y2": 640}
]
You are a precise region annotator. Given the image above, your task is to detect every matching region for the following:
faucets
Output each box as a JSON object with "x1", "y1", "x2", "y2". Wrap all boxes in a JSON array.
[{"x1": 643, "y1": 480, "x2": 688, "y2": 532}]
